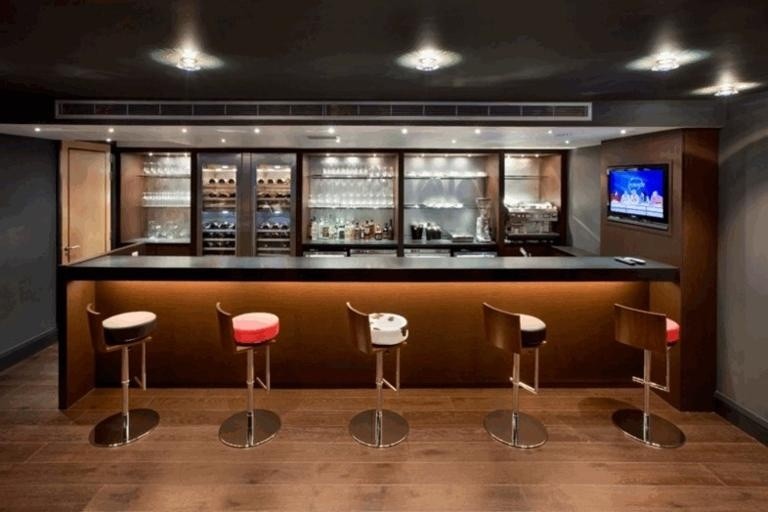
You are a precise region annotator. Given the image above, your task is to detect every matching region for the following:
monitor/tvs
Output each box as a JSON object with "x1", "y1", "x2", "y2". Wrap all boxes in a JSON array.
[{"x1": 606, "y1": 163, "x2": 671, "y2": 230}]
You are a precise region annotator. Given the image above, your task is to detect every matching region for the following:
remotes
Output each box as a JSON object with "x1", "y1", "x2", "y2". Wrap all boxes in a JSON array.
[
  {"x1": 613, "y1": 254, "x2": 636, "y2": 265},
  {"x1": 625, "y1": 256, "x2": 647, "y2": 264}
]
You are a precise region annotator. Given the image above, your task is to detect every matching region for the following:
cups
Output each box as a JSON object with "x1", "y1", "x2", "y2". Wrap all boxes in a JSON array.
[
  {"x1": 142, "y1": 191, "x2": 191, "y2": 207},
  {"x1": 148, "y1": 219, "x2": 191, "y2": 242},
  {"x1": 307, "y1": 168, "x2": 395, "y2": 209},
  {"x1": 143, "y1": 161, "x2": 191, "y2": 178}
]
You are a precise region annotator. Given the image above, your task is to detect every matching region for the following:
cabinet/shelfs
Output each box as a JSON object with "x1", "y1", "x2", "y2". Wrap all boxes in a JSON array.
[{"x1": 112, "y1": 144, "x2": 570, "y2": 256}]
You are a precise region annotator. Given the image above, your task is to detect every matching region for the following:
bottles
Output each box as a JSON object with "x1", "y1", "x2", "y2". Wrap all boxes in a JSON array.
[
  {"x1": 407, "y1": 219, "x2": 443, "y2": 241},
  {"x1": 202, "y1": 177, "x2": 291, "y2": 251},
  {"x1": 310, "y1": 215, "x2": 394, "y2": 241}
]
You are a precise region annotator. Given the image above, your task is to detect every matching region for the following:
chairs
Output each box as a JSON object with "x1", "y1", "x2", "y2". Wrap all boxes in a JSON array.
[
  {"x1": 479, "y1": 301, "x2": 550, "y2": 449},
  {"x1": 342, "y1": 300, "x2": 412, "y2": 451},
  {"x1": 212, "y1": 300, "x2": 282, "y2": 447},
  {"x1": 613, "y1": 304, "x2": 687, "y2": 452},
  {"x1": 83, "y1": 303, "x2": 161, "y2": 451}
]
